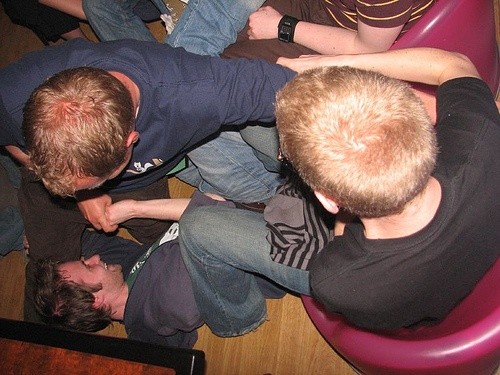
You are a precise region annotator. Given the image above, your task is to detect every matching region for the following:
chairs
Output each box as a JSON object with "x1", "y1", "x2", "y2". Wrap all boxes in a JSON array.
[
  {"x1": 300, "y1": 255, "x2": 500, "y2": 375},
  {"x1": 387, "y1": 0, "x2": 500, "y2": 104}
]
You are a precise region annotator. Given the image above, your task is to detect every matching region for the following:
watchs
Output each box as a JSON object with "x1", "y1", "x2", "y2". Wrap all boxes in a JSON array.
[{"x1": 277, "y1": 15, "x2": 302, "y2": 42}]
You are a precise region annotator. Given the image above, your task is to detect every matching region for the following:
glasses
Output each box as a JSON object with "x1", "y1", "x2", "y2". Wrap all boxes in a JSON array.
[{"x1": 277, "y1": 147, "x2": 302, "y2": 176}]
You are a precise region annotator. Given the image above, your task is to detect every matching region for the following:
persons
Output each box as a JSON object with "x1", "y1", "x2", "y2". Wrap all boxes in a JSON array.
[
  {"x1": 0, "y1": 0, "x2": 89, "y2": 44},
  {"x1": 177, "y1": 45, "x2": 500, "y2": 340},
  {"x1": 0, "y1": 36, "x2": 301, "y2": 266},
  {"x1": 222, "y1": 0, "x2": 439, "y2": 69},
  {"x1": 32, "y1": 188, "x2": 271, "y2": 350},
  {"x1": 77, "y1": 0, "x2": 269, "y2": 59}
]
